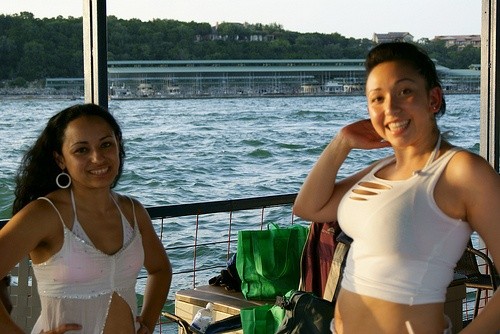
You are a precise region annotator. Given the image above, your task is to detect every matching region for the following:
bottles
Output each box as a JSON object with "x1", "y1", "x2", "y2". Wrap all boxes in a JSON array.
[{"x1": 188, "y1": 302, "x2": 214, "y2": 334}]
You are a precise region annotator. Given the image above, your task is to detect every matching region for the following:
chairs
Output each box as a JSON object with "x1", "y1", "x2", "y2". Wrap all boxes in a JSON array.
[{"x1": 455, "y1": 236, "x2": 500, "y2": 321}]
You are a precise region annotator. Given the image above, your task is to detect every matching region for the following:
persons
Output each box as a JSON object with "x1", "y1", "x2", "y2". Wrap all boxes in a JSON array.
[
  {"x1": 0, "y1": 103, "x2": 172, "y2": 334},
  {"x1": 294, "y1": 42, "x2": 500, "y2": 334}
]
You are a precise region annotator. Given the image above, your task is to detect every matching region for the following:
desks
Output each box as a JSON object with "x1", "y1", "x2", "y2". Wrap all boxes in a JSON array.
[{"x1": 178, "y1": 271, "x2": 470, "y2": 334}]
